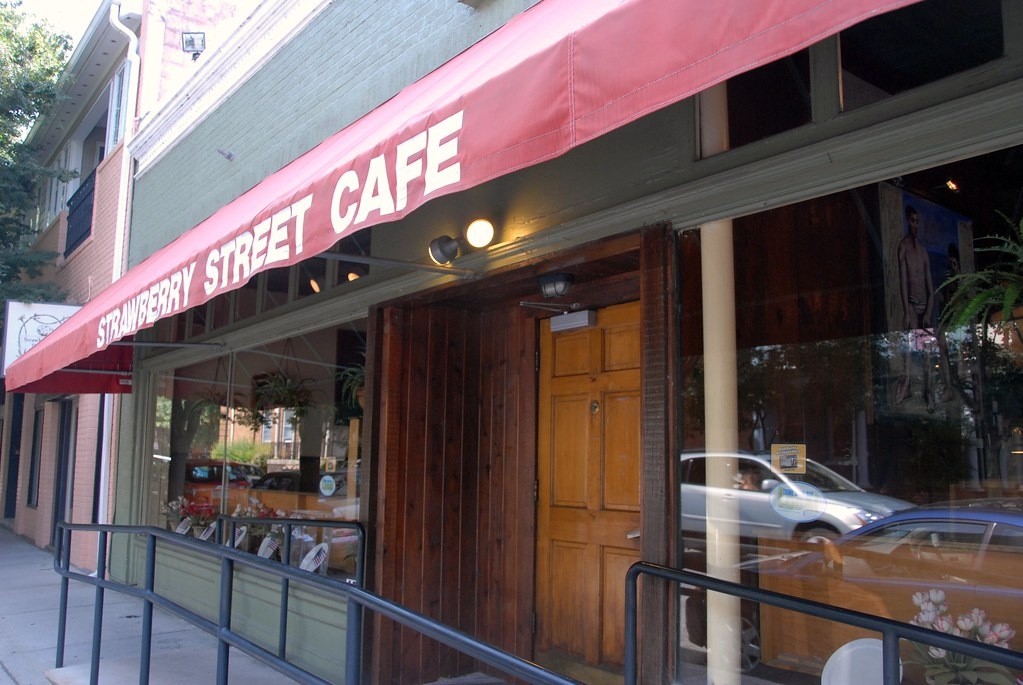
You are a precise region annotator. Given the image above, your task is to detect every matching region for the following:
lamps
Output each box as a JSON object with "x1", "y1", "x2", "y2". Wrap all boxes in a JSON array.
[
  {"x1": 426, "y1": 214, "x2": 496, "y2": 264},
  {"x1": 347, "y1": 264, "x2": 369, "y2": 281},
  {"x1": 309, "y1": 272, "x2": 325, "y2": 293},
  {"x1": 182, "y1": 31, "x2": 206, "y2": 60},
  {"x1": 537, "y1": 272, "x2": 574, "y2": 299}
]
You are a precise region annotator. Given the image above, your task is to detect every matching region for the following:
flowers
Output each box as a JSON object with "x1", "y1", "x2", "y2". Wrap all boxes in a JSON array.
[
  {"x1": 158, "y1": 494, "x2": 308, "y2": 546},
  {"x1": 898, "y1": 588, "x2": 1016, "y2": 685}
]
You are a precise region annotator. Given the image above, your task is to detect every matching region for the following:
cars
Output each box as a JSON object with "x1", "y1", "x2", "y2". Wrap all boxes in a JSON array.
[
  {"x1": 152, "y1": 455, "x2": 361, "y2": 575},
  {"x1": 685, "y1": 499, "x2": 1023, "y2": 685}
]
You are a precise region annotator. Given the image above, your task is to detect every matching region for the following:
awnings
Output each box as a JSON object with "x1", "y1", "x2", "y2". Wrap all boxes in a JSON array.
[{"x1": 6, "y1": 0, "x2": 919, "y2": 395}]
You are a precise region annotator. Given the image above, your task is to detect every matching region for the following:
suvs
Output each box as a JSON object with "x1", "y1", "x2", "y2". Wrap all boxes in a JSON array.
[{"x1": 679, "y1": 449, "x2": 919, "y2": 553}]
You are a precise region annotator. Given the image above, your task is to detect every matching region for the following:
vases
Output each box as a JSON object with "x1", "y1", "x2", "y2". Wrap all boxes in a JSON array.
[
  {"x1": 190, "y1": 526, "x2": 206, "y2": 538},
  {"x1": 925, "y1": 661, "x2": 1017, "y2": 685},
  {"x1": 169, "y1": 522, "x2": 181, "y2": 531}
]
